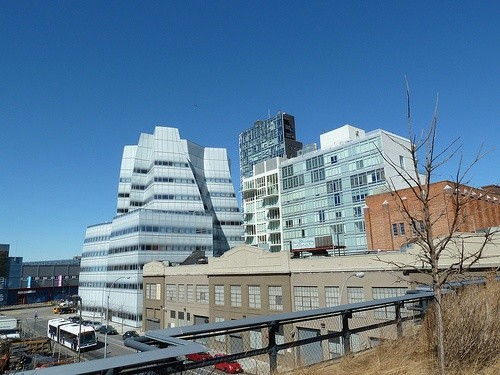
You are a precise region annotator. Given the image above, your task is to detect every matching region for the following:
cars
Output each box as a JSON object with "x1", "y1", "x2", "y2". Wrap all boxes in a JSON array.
[
  {"x1": 98, "y1": 325, "x2": 117, "y2": 336},
  {"x1": 212, "y1": 353, "x2": 242, "y2": 374},
  {"x1": 185, "y1": 351, "x2": 214, "y2": 363},
  {"x1": 54, "y1": 302, "x2": 77, "y2": 314},
  {"x1": 122, "y1": 331, "x2": 139, "y2": 340},
  {"x1": 67, "y1": 314, "x2": 102, "y2": 330}
]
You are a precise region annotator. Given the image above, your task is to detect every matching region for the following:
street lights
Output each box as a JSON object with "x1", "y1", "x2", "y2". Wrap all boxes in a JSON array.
[{"x1": 104, "y1": 276, "x2": 132, "y2": 359}]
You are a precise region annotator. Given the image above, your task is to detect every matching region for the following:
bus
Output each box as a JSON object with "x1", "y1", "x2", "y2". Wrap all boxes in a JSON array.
[{"x1": 47, "y1": 317, "x2": 100, "y2": 353}]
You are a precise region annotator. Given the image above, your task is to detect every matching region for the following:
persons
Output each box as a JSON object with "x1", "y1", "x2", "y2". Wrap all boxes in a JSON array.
[{"x1": 34, "y1": 310, "x2": 39, "y2": 319}]
